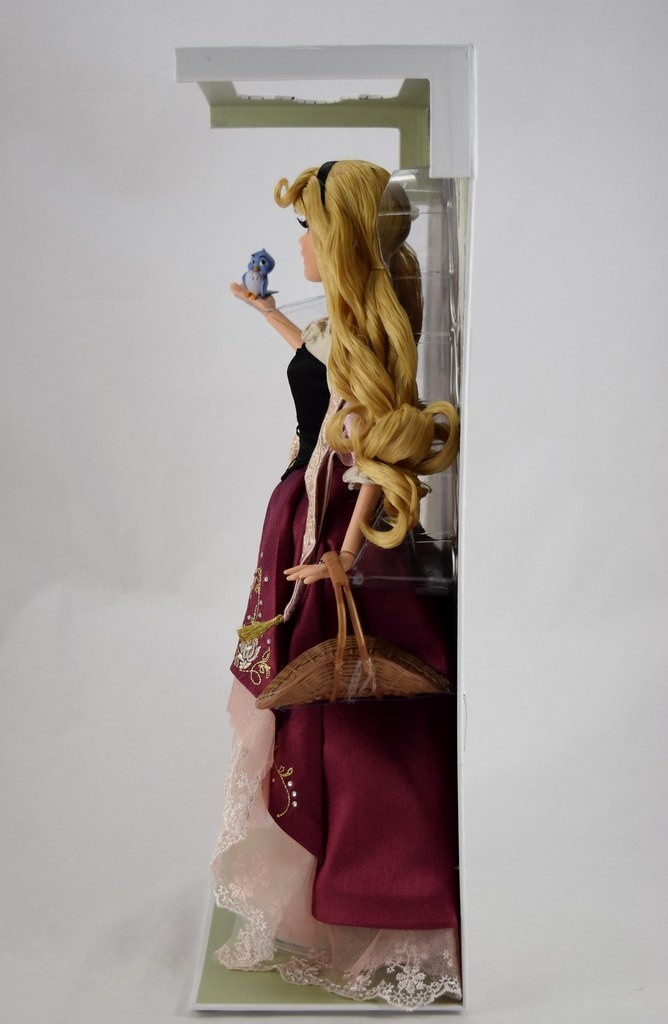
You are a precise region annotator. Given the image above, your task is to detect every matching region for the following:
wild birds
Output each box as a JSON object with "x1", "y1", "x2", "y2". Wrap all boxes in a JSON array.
[{"x1": 242, "y1": 247, "x2": 279, "y2": 301}]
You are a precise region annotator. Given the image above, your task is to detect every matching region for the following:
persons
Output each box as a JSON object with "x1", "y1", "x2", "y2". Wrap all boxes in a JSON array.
[{"x1": 209, "y1": 156, "x2": 462, "y2": 999}]
